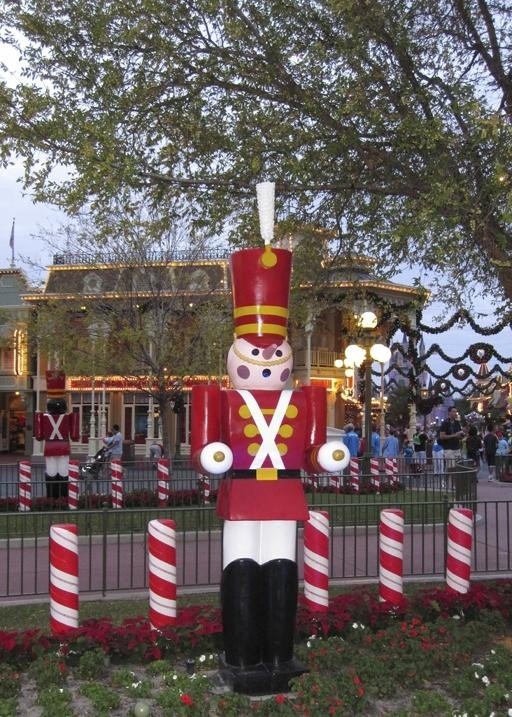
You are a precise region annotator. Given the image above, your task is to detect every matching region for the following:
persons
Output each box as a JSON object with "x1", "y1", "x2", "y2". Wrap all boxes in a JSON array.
[
  {"x1": 343, "y1": 423, "x2": 359, "y2": 457},
  {"x1": 104, "y1": 424, "x2": 123, "y2": 478},
  {"x1": 372, "y1": 407, "x2": 509, "y2": 490}
]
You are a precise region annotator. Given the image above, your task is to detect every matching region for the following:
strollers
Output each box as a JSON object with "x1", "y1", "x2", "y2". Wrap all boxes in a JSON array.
[{"x1": 79, "y1": 446, "x2": 108, "y2": 479}]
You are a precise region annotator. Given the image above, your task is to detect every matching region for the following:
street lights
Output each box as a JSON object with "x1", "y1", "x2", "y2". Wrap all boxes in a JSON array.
[
  {"x1": 419, "y1": 383, "x2": 428, "y2": 436},
  {"x1": 345, "y1": 312, "x2": 391, "y2": 493}
]
